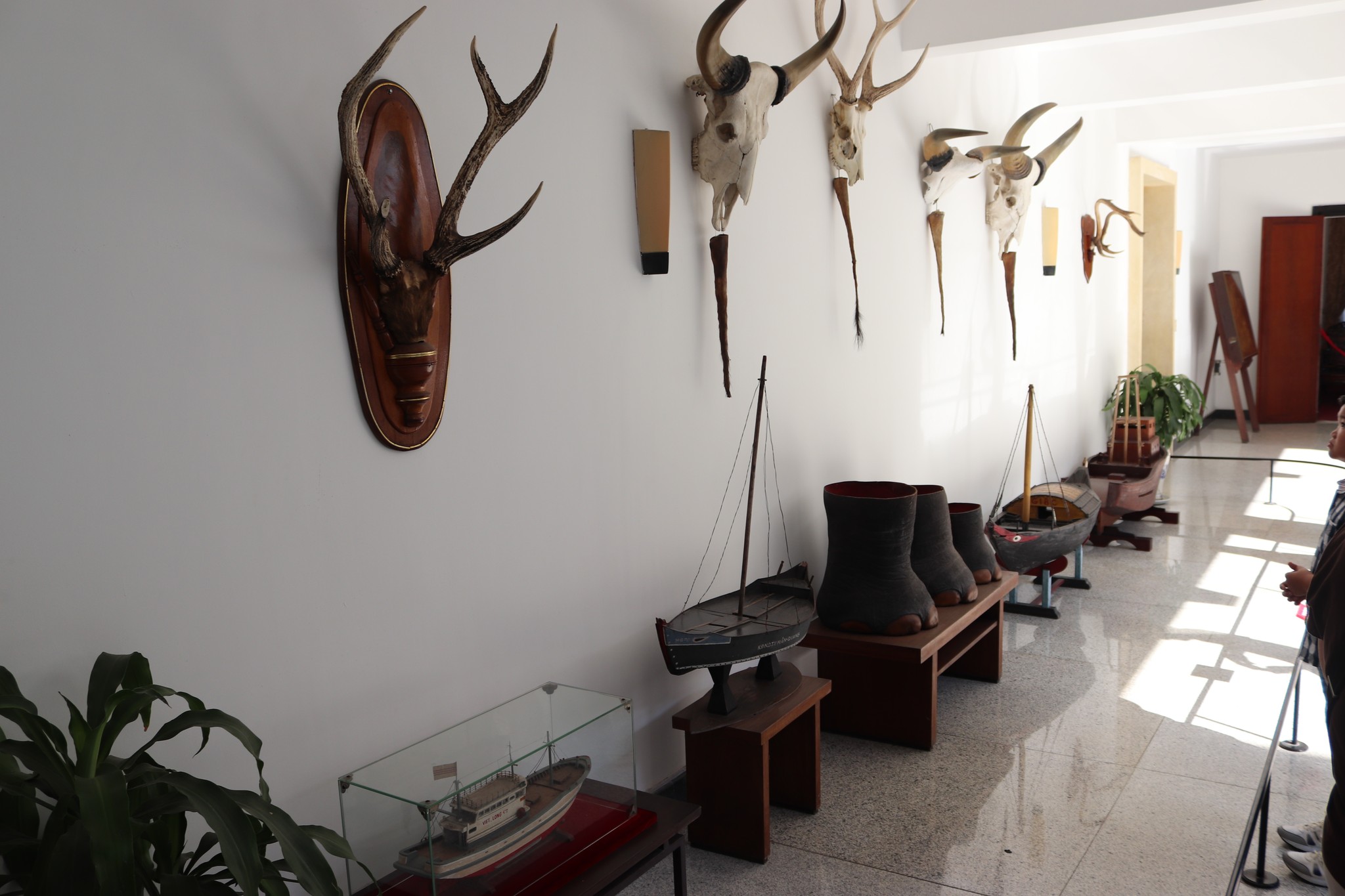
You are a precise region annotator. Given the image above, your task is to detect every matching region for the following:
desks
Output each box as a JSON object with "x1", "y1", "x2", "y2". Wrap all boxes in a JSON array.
[
  {"x1": 793, "y1": 571, "x2": 1022, "y2": 751},
  {"x1": 342, "y1": 778, "x2": 702, "y2": 896},
  {"x1": 672, "y1": 678, "x2": 835, "y2": 864}
]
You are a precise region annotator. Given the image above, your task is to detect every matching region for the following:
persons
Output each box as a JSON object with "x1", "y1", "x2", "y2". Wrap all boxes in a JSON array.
[{"x1": 1277, "y1": 404, "x2": 1345, "y2": 896}]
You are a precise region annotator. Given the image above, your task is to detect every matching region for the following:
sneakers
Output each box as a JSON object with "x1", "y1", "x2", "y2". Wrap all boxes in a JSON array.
[
  {"x1": 1282, "y1": 850, "x2": 1328, "y2": 886},
  {"x1": 1277, "y1": 821, "x2": 1324, "y2": 851}
]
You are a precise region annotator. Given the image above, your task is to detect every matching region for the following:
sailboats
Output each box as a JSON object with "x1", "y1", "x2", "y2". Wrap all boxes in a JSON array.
[
  {"x1": 984, "y1": 383, "x2": 1102, "y2": 573},
  {"x1": 655, "y1": 355, "x2": 816, "y2": 677}
]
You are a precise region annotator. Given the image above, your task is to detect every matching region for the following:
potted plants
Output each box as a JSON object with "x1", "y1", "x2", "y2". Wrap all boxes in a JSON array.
[{"x1": 1101, "y1": 364, "x2": 1203, "y2": 500}]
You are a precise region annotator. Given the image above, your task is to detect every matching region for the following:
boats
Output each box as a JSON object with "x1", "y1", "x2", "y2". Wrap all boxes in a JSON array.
[
  {"x1": 392, "y1": 731, "x2": 592, "y2": 881},
  {"x1": 1060, "y1": 375, "x2": 1170, "y2": 518}
]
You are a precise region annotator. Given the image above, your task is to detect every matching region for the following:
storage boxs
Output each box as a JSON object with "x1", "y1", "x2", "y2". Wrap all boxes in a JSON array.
[{"x1": 337, "y1": 685, "x2": 637, "y2": 896}]
